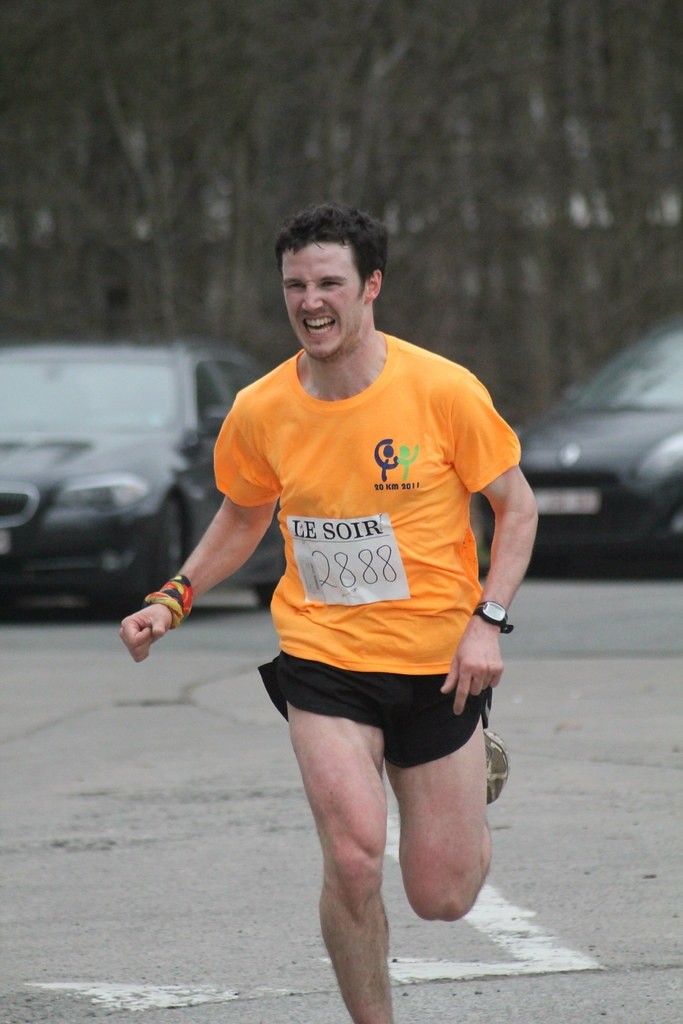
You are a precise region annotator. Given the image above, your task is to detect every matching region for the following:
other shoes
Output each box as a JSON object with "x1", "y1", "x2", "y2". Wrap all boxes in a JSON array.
[{"x1": 482, "y1": 729, "x2": 511, "y2": 804}]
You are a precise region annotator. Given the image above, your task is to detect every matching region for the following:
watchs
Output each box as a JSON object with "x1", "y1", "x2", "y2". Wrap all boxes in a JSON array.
[{"x1": 472, "y1": 601, "x2": 515, "y2": 634}]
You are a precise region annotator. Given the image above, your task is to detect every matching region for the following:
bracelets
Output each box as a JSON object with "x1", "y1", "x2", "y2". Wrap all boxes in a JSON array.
[{"x1": 143, "y1": 573, "x2": 193, "y2": 630}]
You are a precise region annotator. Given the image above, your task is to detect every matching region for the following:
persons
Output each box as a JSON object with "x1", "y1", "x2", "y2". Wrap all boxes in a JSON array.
[{"x1": 117, "y1": 200, "x2": 542, "y2": 1024}]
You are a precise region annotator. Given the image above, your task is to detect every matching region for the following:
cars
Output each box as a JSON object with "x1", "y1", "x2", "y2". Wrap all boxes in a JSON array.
[
  {"x1": 2, "y1": 342, "x2": 293, "y2": 622},
  {"x1": 484, "y1": 319, "x2": 682, "y2": 561}
]
